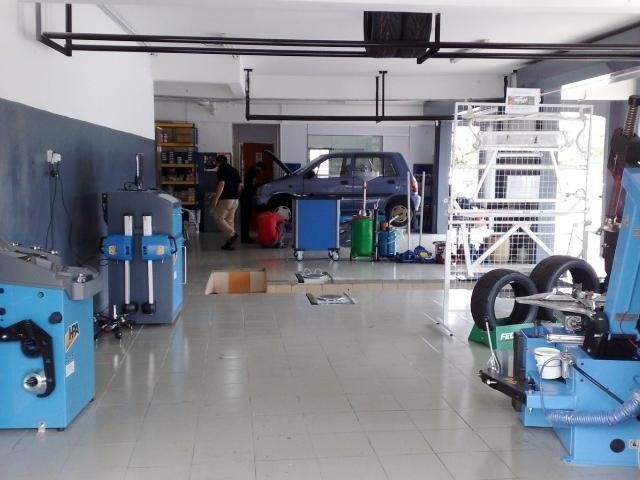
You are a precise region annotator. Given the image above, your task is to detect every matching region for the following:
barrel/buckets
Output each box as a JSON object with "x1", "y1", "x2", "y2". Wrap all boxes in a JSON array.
[
  {"x1": 534, "y1": 347, "x2": 562, "y2": 379},
  {"x1": 352, "y1": 218, "x2": 374, "y2": 256},
  {"x1": 487, "y1": 233, "x2": 510, "y2": 263},
  {"x1": 377, "y1": 230, "x2": 396, "y2": 255}
]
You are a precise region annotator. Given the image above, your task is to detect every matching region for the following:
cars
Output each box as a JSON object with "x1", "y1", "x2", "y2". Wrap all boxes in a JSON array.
[{"x1": 253, "y1": 147, "x2": 421, "y2": 228}]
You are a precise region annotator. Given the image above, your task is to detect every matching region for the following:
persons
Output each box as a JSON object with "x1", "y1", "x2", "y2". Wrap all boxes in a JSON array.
[
  {"x1": 245, "y1": 161, "x2": 267, "y2": 188},
  {"x1": 210, "y1": 155, "x2": 243, "y2": 250},
  {"x1": 240, "y1": 181, "x2": 271, "y2": 244},
  {"x1": 255, "y1": 206, "x2": 288, "y2": 248}
]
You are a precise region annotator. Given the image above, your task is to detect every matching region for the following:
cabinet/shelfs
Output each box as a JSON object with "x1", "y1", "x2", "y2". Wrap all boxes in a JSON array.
[{"x1": 155, "y1": 120, "x2": 199, "y2": 207}]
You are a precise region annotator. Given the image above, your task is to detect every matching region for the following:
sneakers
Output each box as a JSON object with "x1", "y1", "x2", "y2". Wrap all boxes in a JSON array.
[{"x1": 222, "y1": 233, "x2": 239, "y2": 250}]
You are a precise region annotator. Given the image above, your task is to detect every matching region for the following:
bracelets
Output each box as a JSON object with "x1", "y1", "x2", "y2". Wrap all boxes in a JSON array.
[{"x1": 213, "y1": 198, "x2": 218, "y2": 200}]
